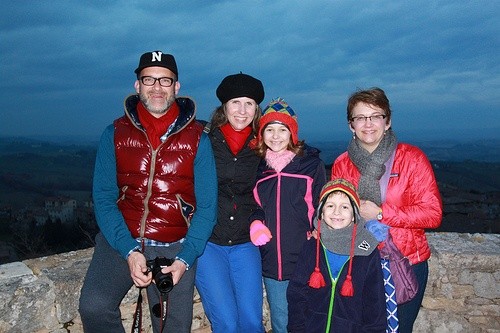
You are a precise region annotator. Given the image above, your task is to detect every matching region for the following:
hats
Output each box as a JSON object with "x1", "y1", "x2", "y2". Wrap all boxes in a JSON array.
[
  {"x1": 215, "y1": 71, "x2": 265, "y2": 105},
  {"x1": 308, "y1": 178, "x2": 360, "y2": 296},
  {"x1": 135, "y1": 51, "x2": 178, "y2": 80},
  {"x1": 248, "y1": 96, "x2": 298, "y2": 149}
]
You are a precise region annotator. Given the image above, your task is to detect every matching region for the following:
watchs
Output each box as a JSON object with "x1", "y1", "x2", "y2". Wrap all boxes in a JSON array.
[{"x1": 376, "y1": 208, "x2": 383, "y2": 221}]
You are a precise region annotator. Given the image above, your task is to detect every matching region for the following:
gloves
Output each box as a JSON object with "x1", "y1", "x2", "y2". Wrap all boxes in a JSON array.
[{"x1": 249, "y1": 221, "x2": 272, "y2": 247}]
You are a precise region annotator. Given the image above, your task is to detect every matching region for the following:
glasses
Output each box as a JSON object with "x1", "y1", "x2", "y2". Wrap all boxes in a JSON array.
[
  {"x1": 140, "y1": 75, "x2": 174, "y2": 87},
  {"x1": 351, "y1": 114, "x2": 385, "y2": 122}
]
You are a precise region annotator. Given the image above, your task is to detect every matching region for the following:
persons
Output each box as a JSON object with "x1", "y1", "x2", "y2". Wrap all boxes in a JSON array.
[
  {"x1": 79, "y1": 50, "x2": 219, "y2": 333},
  {"x1": 193, "y1": 71, "x2": 267, "y2": 333},
  {"x1": 330, "y1": 87, "x2": 443, "y2": 333},
  {"x1": 286, "y1": 177, "x2": 387, "y2": 333},
  {"x1": 246, "y1": 95, "x2": 328, "y2": 333}
]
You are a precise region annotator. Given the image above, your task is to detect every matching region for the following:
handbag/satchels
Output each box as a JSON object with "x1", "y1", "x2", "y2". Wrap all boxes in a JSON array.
[
  {"x1": 380, "y1": 234, "x2": 419, "y2": 304},
  {"x1": 380, "y1": 259, "x2": 399, "y2": 333}
]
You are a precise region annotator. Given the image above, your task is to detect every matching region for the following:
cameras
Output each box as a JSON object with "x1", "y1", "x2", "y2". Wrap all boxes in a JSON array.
[{"x1": 144, "y1": 256, "x2": 173, "y2": 293}]
